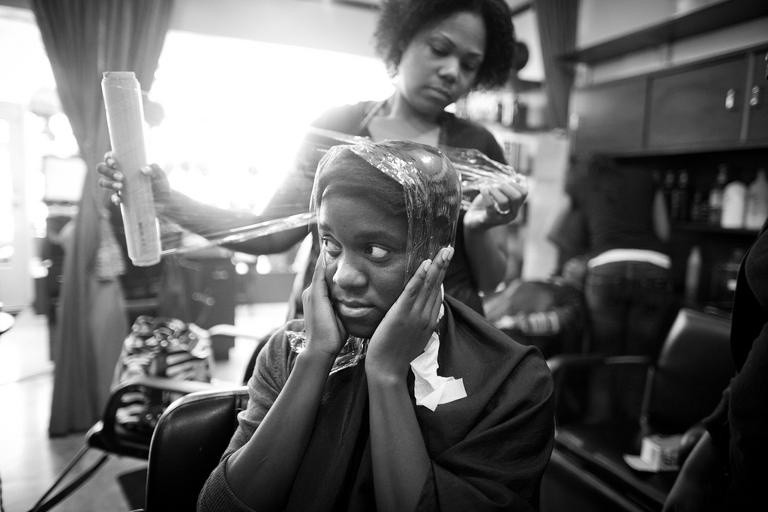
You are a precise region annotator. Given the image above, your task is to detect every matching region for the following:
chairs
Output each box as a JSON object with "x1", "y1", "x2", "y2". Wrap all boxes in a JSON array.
[{"x1": 142, "y1": 386, "x2": 250, "y2": 511}]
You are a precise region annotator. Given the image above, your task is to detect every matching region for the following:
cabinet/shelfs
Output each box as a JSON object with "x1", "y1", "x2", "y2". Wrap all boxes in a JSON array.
[
  {"x1": 564, "y1": 76, "x2": 645, "y2": 156},
  {"x1": 641, "y1": 50, "x2": 767, "y2": 150}
]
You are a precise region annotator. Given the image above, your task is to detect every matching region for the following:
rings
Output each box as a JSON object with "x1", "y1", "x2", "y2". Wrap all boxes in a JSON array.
[{"x1": 494, "y1": 203, "x2": 511, "y2": 216}]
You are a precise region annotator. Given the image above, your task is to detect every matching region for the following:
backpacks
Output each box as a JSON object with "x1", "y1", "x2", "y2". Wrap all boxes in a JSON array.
[{"x1": 106, "y1": 315, "x2": 214, "y2": 440}]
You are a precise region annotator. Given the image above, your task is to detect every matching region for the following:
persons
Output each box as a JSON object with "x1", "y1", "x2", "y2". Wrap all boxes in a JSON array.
[
  {"x1": 201, "y1": 141, "x2": 554, "y2": 511},
  {"x1": 96, "y1": 1, "x2": 525, "y2": 321},
  {"x1": 662, "y1": 216, "x2": 768, "y2": 512},
  {"x1": 566, "y1": 147, "x2": 676, "y2": 426}
]
